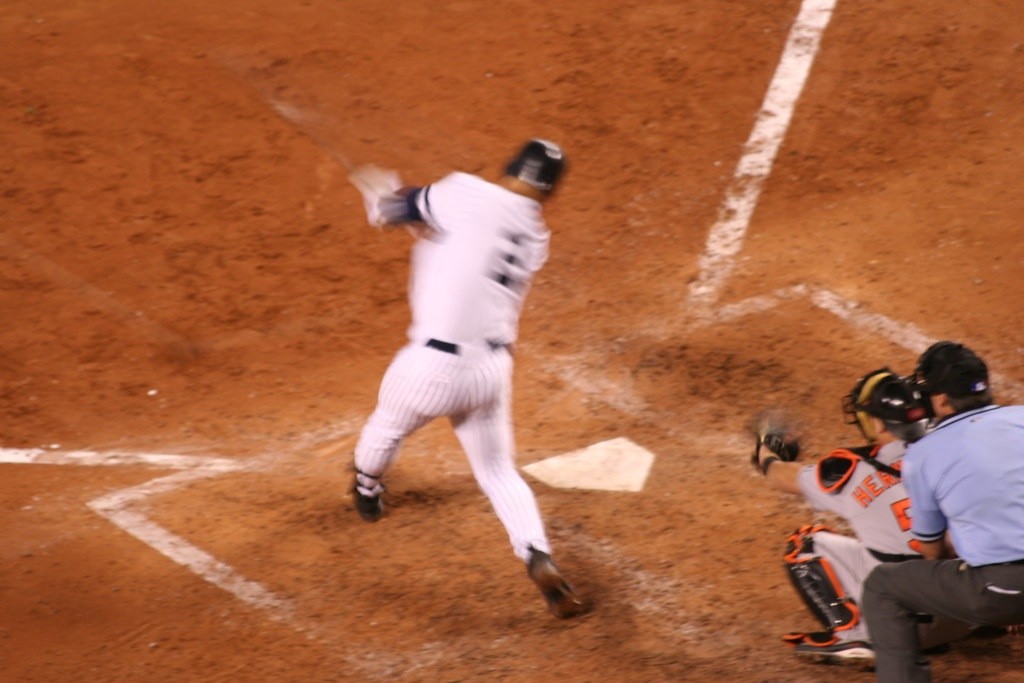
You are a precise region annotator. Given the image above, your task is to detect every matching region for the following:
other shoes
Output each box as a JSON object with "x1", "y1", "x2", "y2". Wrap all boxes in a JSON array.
[
  {"x1": 524, "y1": 547, "x2": 581, "y2": 619},
  {"x1": 353, "y1": 479, "x2": 385, "y2": 519}
]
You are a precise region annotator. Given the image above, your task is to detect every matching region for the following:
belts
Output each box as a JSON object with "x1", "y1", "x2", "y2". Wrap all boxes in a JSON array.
[
  {"x1": 427, "y1": 339, "x2": 498, "y2": 354},
  {"x1": 867, "y1": 547, "x2": 925, "y2": 562}
]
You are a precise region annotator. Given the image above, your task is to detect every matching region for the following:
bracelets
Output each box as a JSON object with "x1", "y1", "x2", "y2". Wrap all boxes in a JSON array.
[{"x1": 761, "y1": 455, "x2": 780, "y2": 475}]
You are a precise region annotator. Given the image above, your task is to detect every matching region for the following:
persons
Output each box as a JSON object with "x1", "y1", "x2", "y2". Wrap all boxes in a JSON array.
[
  {"x1": 863, "y1": 340, "x2": 1024, "y2": 683},
  {"x1": 349, "y1": 140, "x2": 590, "y2": 617},
  {"x1": 757, "y1": 367, "x2": 970, "y2": 664}
]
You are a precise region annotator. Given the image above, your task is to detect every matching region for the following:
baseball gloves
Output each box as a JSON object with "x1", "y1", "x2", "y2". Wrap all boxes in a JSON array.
[{"x1": 745, "y1": 410, "x2": 800, "y2": 472}]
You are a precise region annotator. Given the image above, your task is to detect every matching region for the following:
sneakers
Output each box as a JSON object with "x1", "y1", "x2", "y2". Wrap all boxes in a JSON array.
[{"x1": 793, "y1": 624, "x2": 877, "y2": 667}]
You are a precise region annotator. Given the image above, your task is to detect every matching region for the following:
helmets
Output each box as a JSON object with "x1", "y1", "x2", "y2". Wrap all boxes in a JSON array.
[{"x1": 841, "y1": 368, "x2": 902, "y2": 425}]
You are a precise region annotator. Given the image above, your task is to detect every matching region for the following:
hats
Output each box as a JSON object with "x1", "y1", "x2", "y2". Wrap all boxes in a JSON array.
[
  {"x1": 505, "y1": 139, "x2": 564, "y2": 191},
  {"x1": 902, "y1": 340, "x2": 963, "y2": 386},
  {"x1": 919, "y1": 348, "x2": 990, "y2": 393}
]
